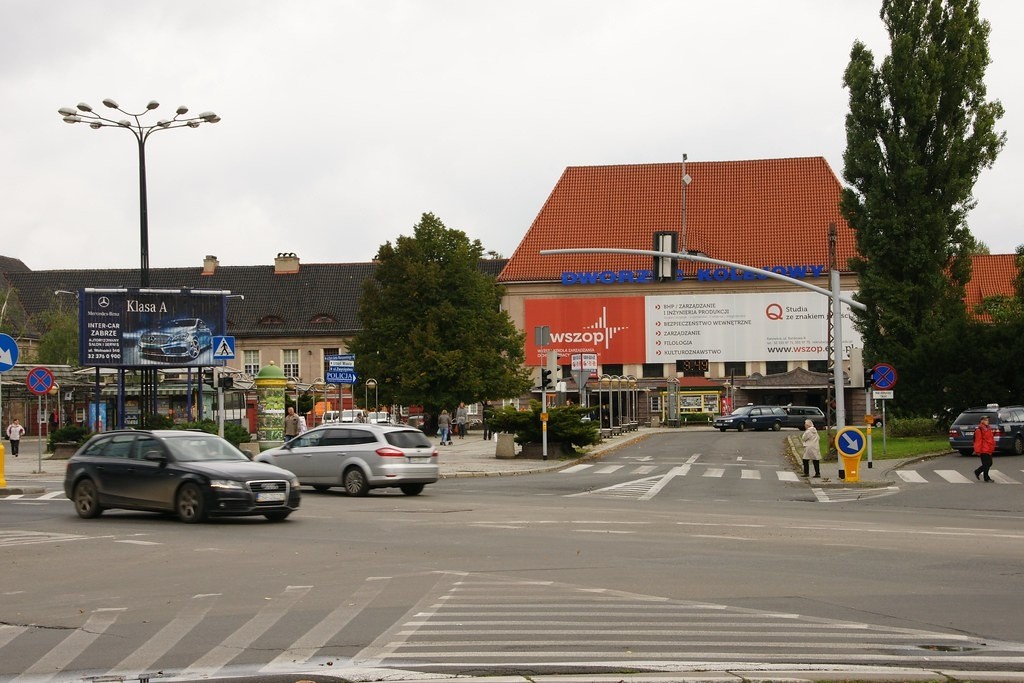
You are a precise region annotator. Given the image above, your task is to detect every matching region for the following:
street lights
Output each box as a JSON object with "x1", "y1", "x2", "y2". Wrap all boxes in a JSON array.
[{"x1": 58, "y1": 100, "x2": 221, "y2": 289}]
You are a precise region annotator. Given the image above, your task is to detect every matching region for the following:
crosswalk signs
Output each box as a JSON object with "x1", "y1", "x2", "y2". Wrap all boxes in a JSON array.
[{"x1": 214, "y1": 336, "x2": 235, "y2": 359}]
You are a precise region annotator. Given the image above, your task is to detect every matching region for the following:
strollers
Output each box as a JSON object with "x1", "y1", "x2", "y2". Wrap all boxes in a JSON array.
[{"x1": 440, "y1": 427, "x2": 453, "y2": 445}]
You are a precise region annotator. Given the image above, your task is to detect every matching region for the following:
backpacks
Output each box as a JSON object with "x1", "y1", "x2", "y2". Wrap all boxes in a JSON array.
[{"x1": 973, "y1": 426, "x2": 982, "y2": 442}]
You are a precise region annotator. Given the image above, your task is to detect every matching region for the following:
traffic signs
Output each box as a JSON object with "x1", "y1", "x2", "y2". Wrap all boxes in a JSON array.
[{"x1": 325, "y1": 372, "x2": 358, "y2": 385}]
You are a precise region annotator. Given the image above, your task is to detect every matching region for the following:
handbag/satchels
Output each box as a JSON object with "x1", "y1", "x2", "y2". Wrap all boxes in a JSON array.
[
  {"x1": 5, "y1": 427, "x2": 11, "y2": 440},
  {"x1": 454, "y1": 424, "x2": 458, "y2": 432},
  {"x1": 437, "y1": 428, "x2": 441, "y2": 434}
]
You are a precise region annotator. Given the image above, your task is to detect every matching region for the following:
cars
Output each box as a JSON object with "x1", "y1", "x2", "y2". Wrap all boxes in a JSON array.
[
  {"x1": 138, "y1": 317, "x2": 213, "y2": 359},
  {"x1": 712, "y1": 405, "x2": 789, "y2": 432},
  {"x1": 871, "y1": 414, "x2": 890, "y2": 428},
  {"x1": 253, "y1": 422, "x2": 439, "y2": 497}
]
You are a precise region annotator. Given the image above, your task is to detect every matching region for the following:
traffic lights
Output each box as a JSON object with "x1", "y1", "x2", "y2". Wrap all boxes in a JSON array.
[
  {"x1": 827, "y1": 368, "x2": 835, "y2": 384},
  {"x1": 205, "y1": 367, "x2": 219, "y2": 390},
  {"x1": 542, "y1": 369, "x2": 552, "y2": 390},
  {"x1": 864, "y1": 368, "x2": 876, "y2": 391},
  {"x1": 220, "y1": 377, "x2": 234, "y2": 388}
]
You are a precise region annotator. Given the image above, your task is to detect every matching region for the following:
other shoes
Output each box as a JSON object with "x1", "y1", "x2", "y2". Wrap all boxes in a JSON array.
[
  {"x1": 985, "y1": 478, "x2": 994, "y2": 483},
  {"x1": 974, "y1": 470, "x2": 980, "y2": 480},
  {"x1": 813, "y1": 475, "x2": 821, "y2": 478},
  {"x1": 801, "y1": 474, "x2": 809, "y2": 477}
]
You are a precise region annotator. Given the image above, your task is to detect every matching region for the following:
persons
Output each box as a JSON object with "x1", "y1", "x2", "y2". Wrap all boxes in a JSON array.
[
  {"x1": 49, "y1": 407, "x2": 58, "y2": 434},
  {"x1": 596, "y1": 403, "x2": 609, "y2": 428},
  {"x1": 483, "y1": 399, "x2": 496, "y2": 440},
  {"x1": 511, "y1": 403, "x2": 517, "y2": 411},
  {"x1": 6, "y1": 419, "x2": 25, "y2": 457},
  {"x1": 801, "y1": 419, "x2": 822, "y2": 479},
  {"x1": 456, "y1": 402, "x2": 468, "y2": 439},
  {"x1": 370, "y1": 404, "x2": 388, "y2": 412},
  {"x1": 438, "y1": 410, "x2": 453, "y2": 446},
  {"x1": 396, "y1": 409, "x2": 402, "y2": 424},
  {"x1": 191, "y1": 402, "x2": 197, "y2": 423},
  {"x1": 354, "y1": 413, "x2": 365, "y2": 423},
  {"x1": 565, "y1": 400, "x2": 575, "y2": 408},
  {"x1": 973, "y1": 414, "x2": 996, "y2": 483},
  {"x1": 282, "y1": 407, "x2": 301, "y2": 446}
]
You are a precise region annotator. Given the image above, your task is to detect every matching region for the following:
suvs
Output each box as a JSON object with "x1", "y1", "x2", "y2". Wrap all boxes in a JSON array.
[
  {"x1": 65, "y1": 428, "x2": 303, "y2": 523},
  {"x1": 949, "y1": 405, "x2": 1024, "y2": 457}
]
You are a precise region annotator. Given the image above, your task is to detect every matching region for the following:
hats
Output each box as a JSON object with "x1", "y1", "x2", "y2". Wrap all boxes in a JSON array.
[{"x1": 980, "y1": 415, "x2": 990, "y2": 420}]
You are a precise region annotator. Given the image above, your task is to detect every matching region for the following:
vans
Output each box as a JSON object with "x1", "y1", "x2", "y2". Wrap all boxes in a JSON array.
[
  {"x1": 778, "y1": 406, "x2": 826, "y2": 431},
  {"x1": 322, "y1": 410, "x2": 390, "y2": 424}
]
